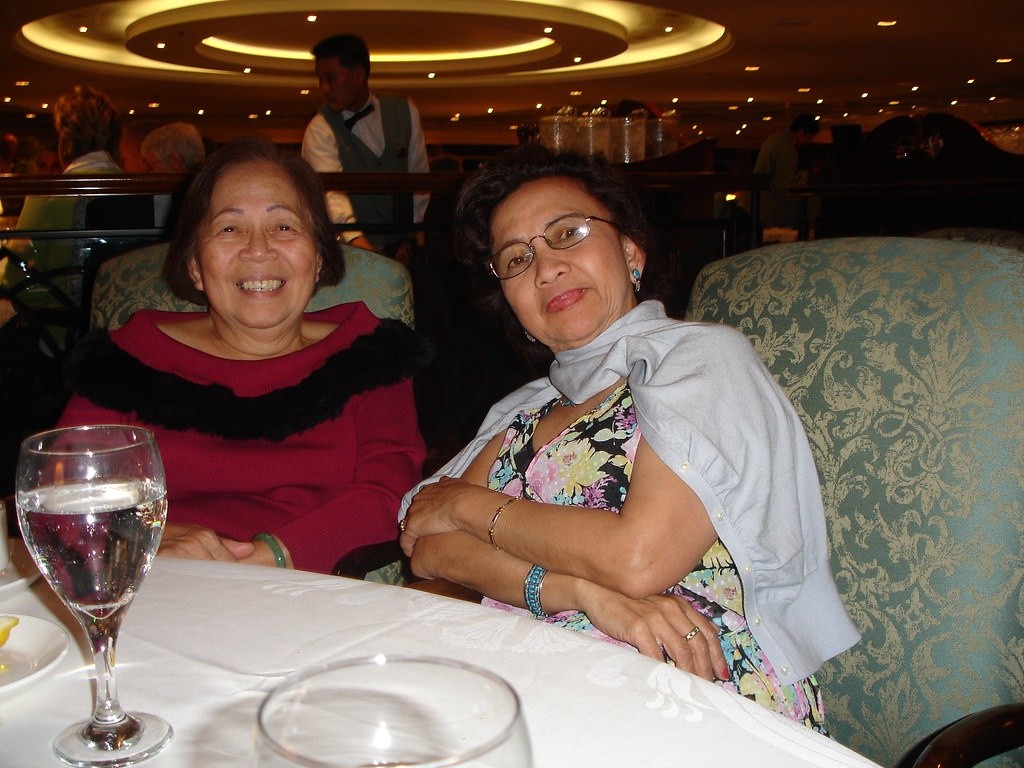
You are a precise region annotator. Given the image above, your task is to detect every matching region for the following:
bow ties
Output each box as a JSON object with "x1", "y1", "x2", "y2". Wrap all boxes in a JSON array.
[{"x1": 344, "y1": 104, "x2": 376, "y2": 132}]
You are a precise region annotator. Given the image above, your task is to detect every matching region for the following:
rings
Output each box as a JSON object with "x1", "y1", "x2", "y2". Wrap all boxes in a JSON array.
[
  {"x1": 683, "y1": 627, "x2": 699, "y2": 641},
  {"x1": 401, "y1": 520, "x2": 405, "y2": 531}
]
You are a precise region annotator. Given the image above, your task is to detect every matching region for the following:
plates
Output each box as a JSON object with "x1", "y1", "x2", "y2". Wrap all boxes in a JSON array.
[
  {"x1": 0, "y1": 615, "x2": 70, "y2": 692},
  {"x1": 0, "y1": 538, "x2": 41, "y2": 590}
]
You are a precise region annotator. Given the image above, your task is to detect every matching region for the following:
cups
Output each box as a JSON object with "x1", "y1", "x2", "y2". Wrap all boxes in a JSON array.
[
  {"x1": 609, "y1": 118, "x2": 645, "y2": 164},
  {"x1": 572, "y1": 118, "x2": 610, "y2": 162},
  {"x1": 540, "y1": 116, "x2": 573, "y2": 154},
  {"x1": 258, "y1": 655, "x2": 532, "y2": 768},
  {"x1": 0, "y1": 501, "x2": 9, "y2": 570}
]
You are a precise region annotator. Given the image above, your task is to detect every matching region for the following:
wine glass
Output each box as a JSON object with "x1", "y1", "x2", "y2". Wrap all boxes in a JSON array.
[{"x1": 14, "y1": 423, "x2": 173, "y2": 768}]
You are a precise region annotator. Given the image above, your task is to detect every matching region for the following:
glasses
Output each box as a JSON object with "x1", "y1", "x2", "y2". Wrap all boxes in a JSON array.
[
  {"x1": 144, "y1": 152, "x2": 164, "y2": 175},
  {"x1": 487, "y1": 212, "x2": 622, "y2": 280}
]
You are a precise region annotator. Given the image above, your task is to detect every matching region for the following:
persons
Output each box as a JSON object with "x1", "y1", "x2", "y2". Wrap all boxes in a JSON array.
[
  {"x1": 400, "y1": 149, "x2": 862, "y2": 740},
  {"x1": 40, "y1": 136, "x2": 426, "y2": 575},
  {"x1": 0, "y1": 85, "x2": 126, "y2": 357},
  {"x1": 302, "y1": 33, "x2": 432, "y2": 265},
  {"x1": 753, "y1": 114, "x2": 820, "y2": 230},
  {"x1": 140, "y1": 123, "x2": 204, "y2": 171}
]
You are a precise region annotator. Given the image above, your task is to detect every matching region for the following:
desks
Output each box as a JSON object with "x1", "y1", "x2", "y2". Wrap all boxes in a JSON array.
[{"x1": 0, "y1": 534, "x2": 885, "y2": 768}]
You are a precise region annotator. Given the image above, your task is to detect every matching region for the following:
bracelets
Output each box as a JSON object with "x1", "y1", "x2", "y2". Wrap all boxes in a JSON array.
[
  {"x1": 489, "y1": 496, "x2": 522, "y2": 549},
  {"x1": 524, "y1": 564, "x2": 548, "y2": 616},
  {"x1": 252, "y1": 532, "x2": 286, "y2": 568}
]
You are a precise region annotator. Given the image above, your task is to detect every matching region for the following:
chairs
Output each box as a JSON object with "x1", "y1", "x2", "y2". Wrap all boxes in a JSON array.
[
  {"x1": 93, "y1": 241, "x2": 415, "y2": 585},
  {"x1": 684, "y1": 236, "x2": 1023, "y2": 768}
]
